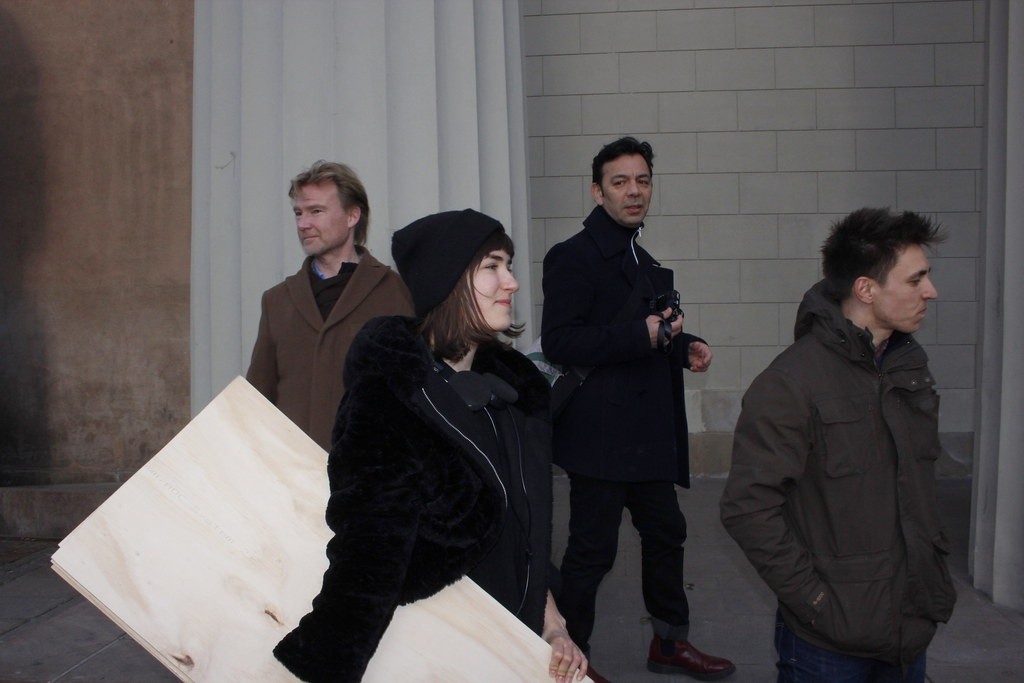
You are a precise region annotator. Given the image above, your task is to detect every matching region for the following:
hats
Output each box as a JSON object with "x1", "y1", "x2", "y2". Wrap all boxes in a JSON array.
[{"x1": 391, "y1": 209, "x2": 503, "y2": 318}]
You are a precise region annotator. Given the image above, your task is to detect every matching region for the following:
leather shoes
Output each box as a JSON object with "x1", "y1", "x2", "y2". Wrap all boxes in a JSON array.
[
  {"x1": 647, "y1": 636, "x2": 736, "y2": 681},
  {"x1": 585, "y1": 664, "x2": 611, "y2": 682}
]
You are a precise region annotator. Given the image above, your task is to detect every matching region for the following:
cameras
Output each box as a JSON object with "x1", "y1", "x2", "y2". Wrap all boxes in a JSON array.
[{"x1": 649, "y1": 288, "x2": 680, "y2": 323}]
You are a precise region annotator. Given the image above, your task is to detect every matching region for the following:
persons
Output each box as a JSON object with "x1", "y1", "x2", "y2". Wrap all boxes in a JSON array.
[
  {"x1": 245, "y1": 162, "x2": 418, "y2": 453},
  {"x1": 272, "y1": 209, "x2": 588, "y2": 683},
  {"x1": 719, "y1": 207, "x2": 959, "y2": 683},
  {"x1": 540, "y1": 138, "x2": 735, "y2": 683}
]
